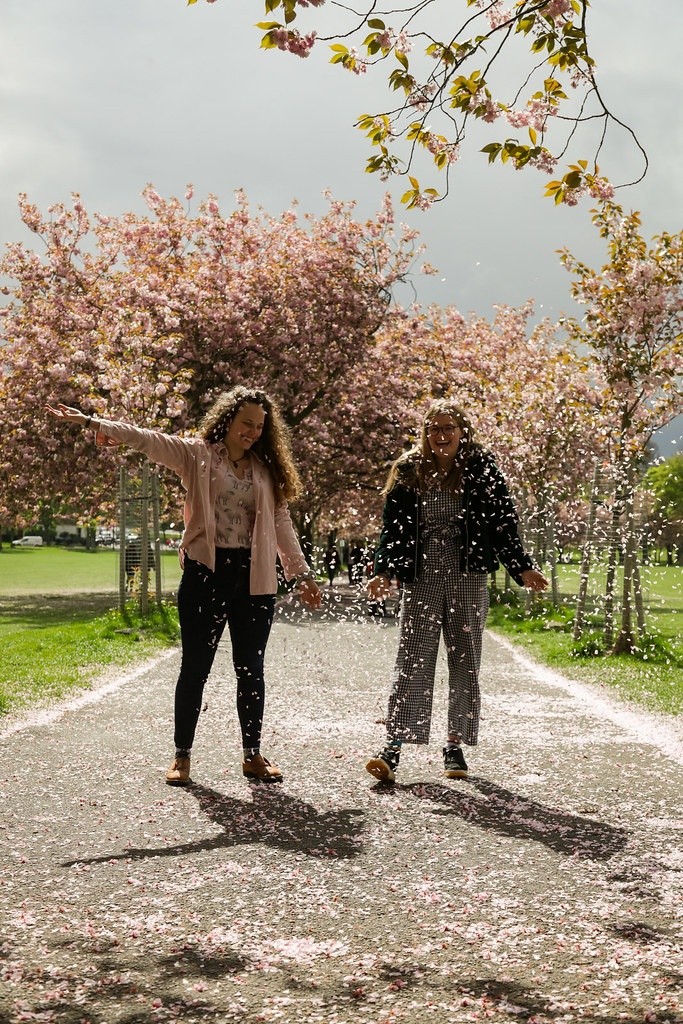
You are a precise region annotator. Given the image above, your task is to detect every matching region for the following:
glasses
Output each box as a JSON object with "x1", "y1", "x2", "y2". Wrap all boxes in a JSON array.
[{"x1": 427, "y1": 424, "x2": 459, "y2": 437}]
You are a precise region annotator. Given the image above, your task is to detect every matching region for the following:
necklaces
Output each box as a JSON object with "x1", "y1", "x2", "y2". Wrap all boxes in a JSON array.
[{"x1": 228, "y1": 454, "x2": 246, "y2": 468}]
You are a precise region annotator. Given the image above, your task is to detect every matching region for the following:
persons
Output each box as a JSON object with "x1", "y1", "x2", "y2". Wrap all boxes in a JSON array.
[
  {"x1": 362, "y1": 401, "x2": 548, "y2": 785},
  {"x1": 325, "y1": 542, "x2": 388, "y2": 615},
  {"x1": 124, "y1": 537, "x2": 157, "y2": 593},
  {"x1": 43, "y1": 384, "x2": 323, "y2": 786}
]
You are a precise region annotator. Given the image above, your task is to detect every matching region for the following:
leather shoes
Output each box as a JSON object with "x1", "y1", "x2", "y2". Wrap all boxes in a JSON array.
[
  {"x1": 243, "y1": 752, "x2": 283, "y2": 783},
  {"x1": 165, "y1": 748, "x2": 191, "y2": 785}
]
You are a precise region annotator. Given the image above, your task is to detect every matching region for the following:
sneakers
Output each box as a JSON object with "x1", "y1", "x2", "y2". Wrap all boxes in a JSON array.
[
  {"x1": 442, "y1": 746, "x2": 468, "y2": 778},
  {"x1": 365, "y1": 747, "x2": 401, "y2": 782}
]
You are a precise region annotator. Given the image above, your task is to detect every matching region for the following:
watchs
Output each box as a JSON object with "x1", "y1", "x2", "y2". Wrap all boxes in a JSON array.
[{"x1": 81, "y1": 415, "x2": 91, "y2": 430}]
